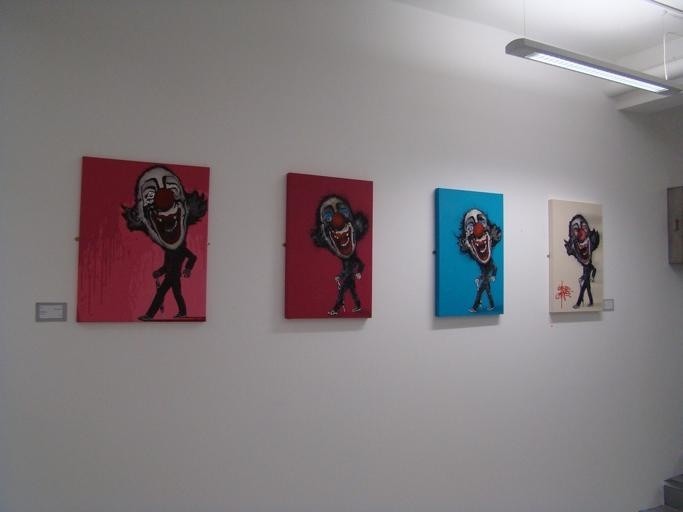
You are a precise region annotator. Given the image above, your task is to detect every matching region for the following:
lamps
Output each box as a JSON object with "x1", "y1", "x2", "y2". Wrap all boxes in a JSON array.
[{"x1": 504, "y1": 0, "x2": 683, "y2": 96}]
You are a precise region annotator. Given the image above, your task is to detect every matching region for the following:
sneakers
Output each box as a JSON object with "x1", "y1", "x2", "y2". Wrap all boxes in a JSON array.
[
  {"x1": 467, "y1": 306, "x2": 497, "y2": 312},
  {"x1": 137, "y1": 312, "x2": 187, "y2": 321},
  {"x1": 327, "y1": 306, "x2": 362, "y2": 316}
]
